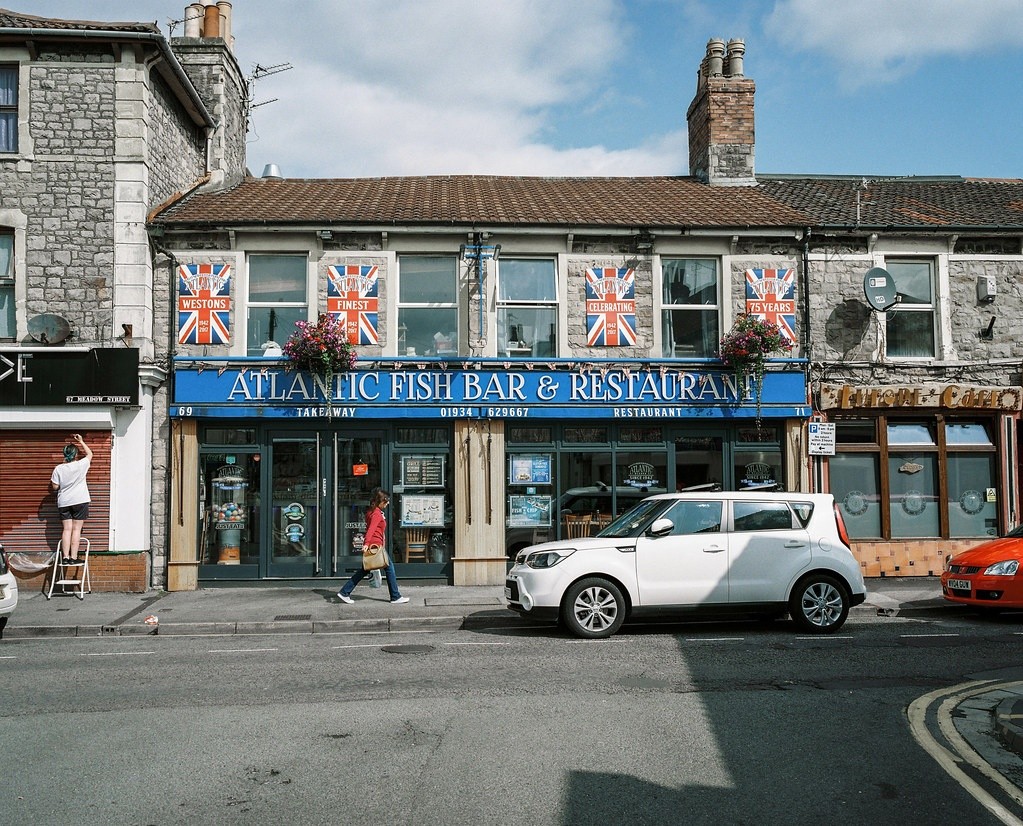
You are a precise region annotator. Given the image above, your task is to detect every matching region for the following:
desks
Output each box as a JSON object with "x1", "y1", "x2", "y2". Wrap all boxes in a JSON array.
[{"x1": 560, "y1": 521, "x2": 612, "y2": 537}]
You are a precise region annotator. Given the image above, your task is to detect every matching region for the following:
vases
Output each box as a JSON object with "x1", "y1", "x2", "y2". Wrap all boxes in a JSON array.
[{"x1": 297, "y1": 356, "x2": 338, "y2": 373}]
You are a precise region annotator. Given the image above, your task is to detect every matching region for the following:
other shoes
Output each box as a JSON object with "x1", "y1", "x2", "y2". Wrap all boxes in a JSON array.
[
  {"x1": 69, "y1": 558, "x2": 84, "y2": 564},
  {"x1": 62, "y1": 558, "x2": 69, "y2": 564}
]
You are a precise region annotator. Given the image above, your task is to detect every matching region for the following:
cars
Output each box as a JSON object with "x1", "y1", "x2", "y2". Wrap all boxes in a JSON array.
[{"x1": 941, "y1": 522, "x2": 1023, "y2": 610}]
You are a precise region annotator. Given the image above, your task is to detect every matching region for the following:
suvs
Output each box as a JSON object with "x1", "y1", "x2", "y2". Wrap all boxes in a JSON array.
[
  {"x1": 504, "y1": 481, "x2": 676, "y2": 561},
  {"x1": 504, "y1": 482, "x2": 867, "y2": 641}
]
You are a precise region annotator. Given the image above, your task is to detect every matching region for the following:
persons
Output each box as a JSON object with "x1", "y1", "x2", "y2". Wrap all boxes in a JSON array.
[
  {"x1": 50, "y1": 434, "x2": 93, "y2": 565},
  {"x1": 336, "y1": 489, "x2": 410, "y2": 604}
]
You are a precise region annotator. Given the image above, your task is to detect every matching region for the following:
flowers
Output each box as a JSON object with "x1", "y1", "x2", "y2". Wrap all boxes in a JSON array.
[
  {"x1": 713, "y1": 311, "x2": 797, "y2": 443},
  {"x1": 277, "y1": 311, "x2": 358, "y2": 373}
]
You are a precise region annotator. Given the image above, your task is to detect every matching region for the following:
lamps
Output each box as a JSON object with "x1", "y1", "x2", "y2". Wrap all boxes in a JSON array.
[
  {"x1": 319, "y1": 230, "x2": 333, "y2": 240},
  {"x1": 633, "y1": 230, "x2": 655, "y2": 249}
]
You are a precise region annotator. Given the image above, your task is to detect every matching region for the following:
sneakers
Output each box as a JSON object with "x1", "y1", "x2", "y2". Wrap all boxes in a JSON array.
[
  {"x1": 337, "y1": 593, "x2": 354, "y2": 604},
  {"x1": 391, "y1": 596, "x2": 409, "y2": 604}
]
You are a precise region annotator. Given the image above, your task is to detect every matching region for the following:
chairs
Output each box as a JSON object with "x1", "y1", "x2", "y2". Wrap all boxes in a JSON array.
[
  {"x1": 566, "y1": 515, "x2": 592, "y2": 540},
  {"x1": 405, "y1": 529, "x2": 429, "y2": 564},
  {"x1": 596, "y1": 515, "x2": 621, "y2": 531}
]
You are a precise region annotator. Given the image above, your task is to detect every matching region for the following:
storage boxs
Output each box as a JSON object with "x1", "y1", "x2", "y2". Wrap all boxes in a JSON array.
[{"x1": 508, "y1": 341, "x2": 519, "y2": 348}]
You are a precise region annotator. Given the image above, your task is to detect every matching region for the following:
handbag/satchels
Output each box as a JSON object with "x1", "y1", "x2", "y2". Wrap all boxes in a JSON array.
[
  {"x1": 367, "y1": 569, "x2": 382, "y2": 588},
  {"x1": 363, "y1": 544, "x2": 390, "y2": 571}
]
